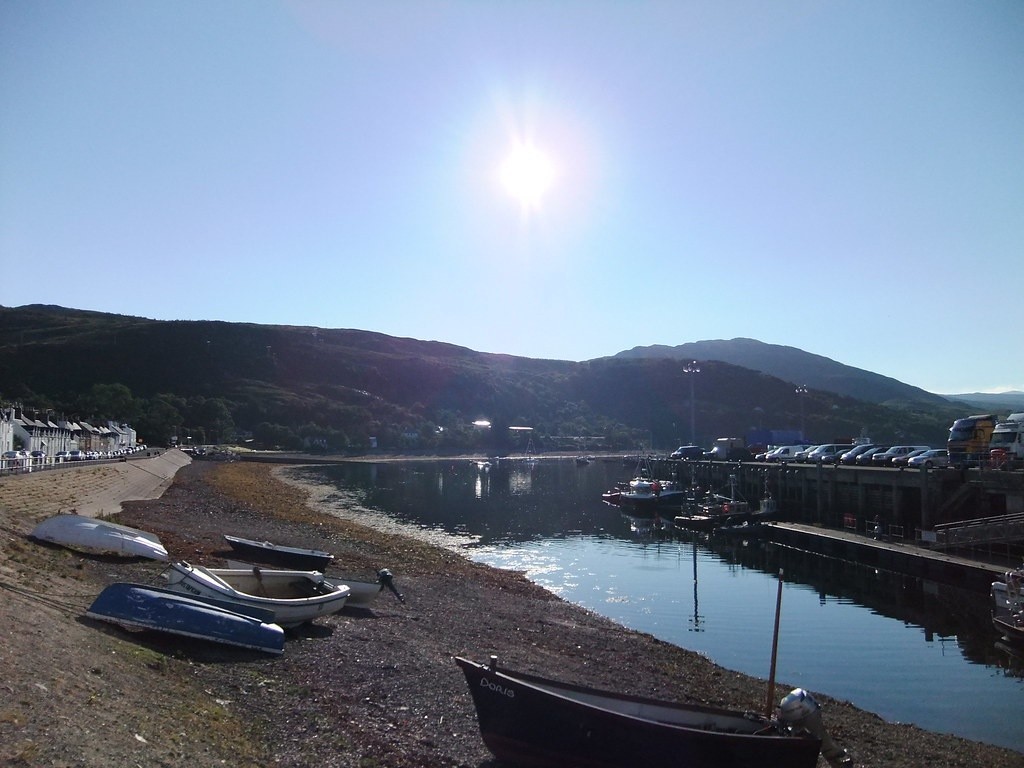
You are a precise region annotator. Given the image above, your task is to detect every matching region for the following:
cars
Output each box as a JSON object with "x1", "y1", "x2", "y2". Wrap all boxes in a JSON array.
[
  {"x1": 54, "y1": 452, "x2": 71, "y2": 462},
  {"x1": 69, "y1": 451, "x2": 85, "y2": 461},
  {"x1": 31, "y1": 451, "x2": 47, "y2": 462},
  {"x1": 85, "y1": 445, "x2": 147, "y2": 460},
  {"x1": 2, "y1": 451, "x2": 27, "y2": 466}
]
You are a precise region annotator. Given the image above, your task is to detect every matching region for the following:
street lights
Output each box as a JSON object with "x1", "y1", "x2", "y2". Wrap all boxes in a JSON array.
[
  {"x1": 683, "y1": 360, "x2": 701, "y2": 445},
  {"x1": 795, "y1": 384, "x2": 808, "y2": 442},
  {"x1": 685, "y1": 540, "x2": 712, "y2": 631}
]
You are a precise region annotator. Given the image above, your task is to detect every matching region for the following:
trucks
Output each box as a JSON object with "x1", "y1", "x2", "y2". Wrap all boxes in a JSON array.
[{"x1": 947, "y1": 413, "x2": 1024, "y2": 469}]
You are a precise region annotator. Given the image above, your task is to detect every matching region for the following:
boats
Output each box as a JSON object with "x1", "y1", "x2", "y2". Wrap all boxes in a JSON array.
[
  {"x1": 212, "y1": 552, "x2": 407, "y2": 615},
  {"x1": 451, "y1": 654, "x2": 822, "y2": 768},
  {"x1": 600, "y1": 480, "x2": 688, "y2": 503},
  {"x1": 169, "y1": 563, "x2": 350, "y2": 629},
  {"x1": 32, "y1": 511, "x2": 168, "y2": 561},
  {"x1": 674, "y1": 480, "x2": 778, "y2": 523},
  {"x1": 85, "y1": 581, "x2": 286, "y2": 656},
  {"x1": 225, "y1": 535, "x2": 333, "y2": 574}
]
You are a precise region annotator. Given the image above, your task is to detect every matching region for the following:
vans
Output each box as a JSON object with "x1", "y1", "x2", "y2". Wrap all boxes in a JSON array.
[
  {"x1": 754, "y1": 444, "x2": 949, "y2": 468},
  {"x1": 670, "y1": 446, "x2": 701, "y2": 460}
]
